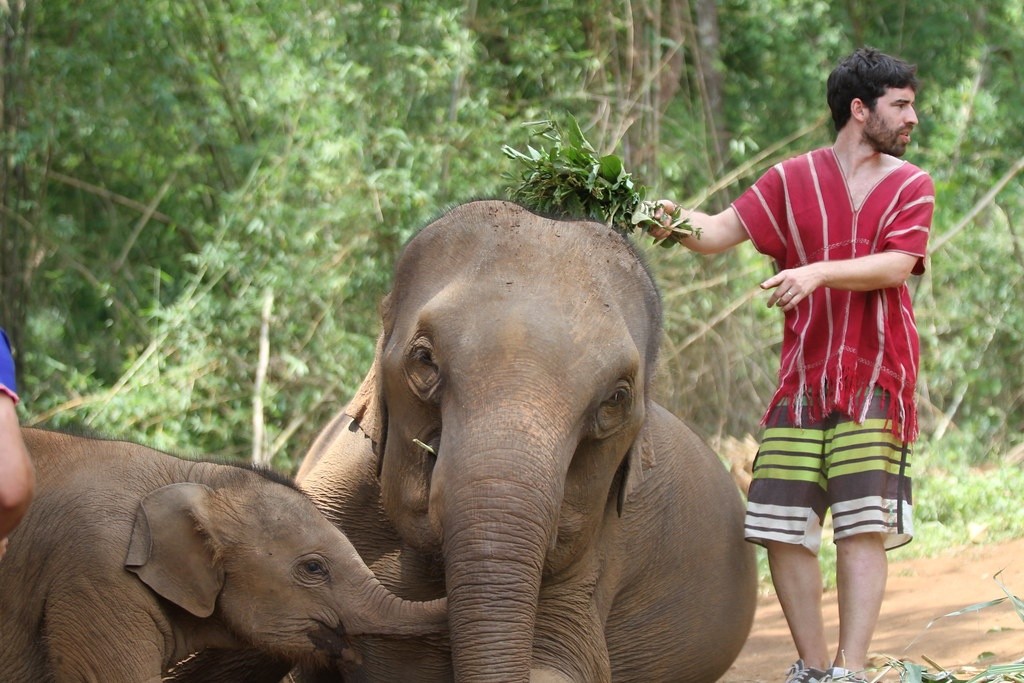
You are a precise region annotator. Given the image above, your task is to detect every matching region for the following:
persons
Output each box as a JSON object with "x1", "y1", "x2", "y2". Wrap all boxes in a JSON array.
[
  {"x1": 0, "y1": 329, "x2": 37, "y2": 561},
  {"x1": 646, "y1": 46, "x2": 935, "y2": 683}
]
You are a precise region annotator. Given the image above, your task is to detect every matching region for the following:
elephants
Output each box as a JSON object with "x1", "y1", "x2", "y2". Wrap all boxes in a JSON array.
[
  {"x1": 291, "y1": 193, "x2": 759, "y2": 683},
  {"x1": 1, "y1": 423, "x2": 450, "y2": 683}
]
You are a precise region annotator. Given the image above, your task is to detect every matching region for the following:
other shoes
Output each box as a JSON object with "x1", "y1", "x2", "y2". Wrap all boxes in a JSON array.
[{"x1": 785, "y1": 658, "x2": 868, "y2": 683}]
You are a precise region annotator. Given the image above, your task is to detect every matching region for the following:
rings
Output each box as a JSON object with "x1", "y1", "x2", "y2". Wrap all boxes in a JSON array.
[{"x1": 787, "y1": 291, "x2": 793, "y2": 298}]
